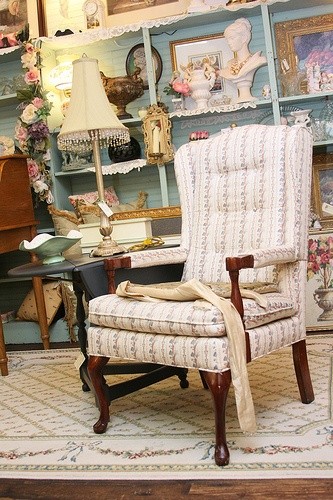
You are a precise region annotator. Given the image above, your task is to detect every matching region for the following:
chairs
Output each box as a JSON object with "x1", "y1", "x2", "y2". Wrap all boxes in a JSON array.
[
  {"x1": 86, "y1": 124, "x2": 318, "y2": 467},
  {"x1": 73, "y1": 241, "x2": 191, "y2": 405}
]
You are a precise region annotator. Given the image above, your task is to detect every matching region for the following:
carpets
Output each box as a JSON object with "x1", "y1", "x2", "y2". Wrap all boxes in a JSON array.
[{"x1": 0, "y1": 332, "x2": 333, "y2": 480}]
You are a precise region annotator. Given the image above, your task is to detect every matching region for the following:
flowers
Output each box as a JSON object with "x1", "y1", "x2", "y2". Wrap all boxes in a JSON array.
[
  {"x1": 12, "y1": 35, "x2": 55, "y2": 206},
  {"x1": 162, "y1": 76, "x2": 193, "y2": 100}
]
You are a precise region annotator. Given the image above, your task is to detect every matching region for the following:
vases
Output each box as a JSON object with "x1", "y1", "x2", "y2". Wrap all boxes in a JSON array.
[
  {"x1": 171, "y1": 97, "x2": 186, "y2": 112},
  {"x1": 184, "y1": 67, "x2": 217, "y2": 109},
  {"x1": 100, "y1": 66, "x2": 144, "y2": 120}
]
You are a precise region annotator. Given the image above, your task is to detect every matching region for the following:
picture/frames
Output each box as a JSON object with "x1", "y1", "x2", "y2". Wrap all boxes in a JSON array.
[
  {"x1": 312, "y1": 162, "x2": 333, "y2": 227},
  {"x1": 125, "y1": 43, "x2": 163, "y2": 90},
  {"x1": 0, "y1": 0, "x2": 46, "y2": 49},
  {"x1": 169, "y1": 32, "x2": 239, "y2": 111},
  {"x1": 274, "y1": 13, "x2": 333, "y2": 98},
  {"x1": 306, "y1": 228, "x2": 333, "y2": 332}
]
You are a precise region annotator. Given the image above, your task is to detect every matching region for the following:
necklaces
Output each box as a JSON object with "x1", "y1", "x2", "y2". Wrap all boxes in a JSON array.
[{"x1": 231, "y1": 53, "x2": 252, "y2": 74}]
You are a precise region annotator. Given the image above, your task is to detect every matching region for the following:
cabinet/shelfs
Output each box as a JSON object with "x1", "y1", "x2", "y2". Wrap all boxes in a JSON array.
[{"x1": 0, "y1": 0, "x2": 333, "y2": 345}]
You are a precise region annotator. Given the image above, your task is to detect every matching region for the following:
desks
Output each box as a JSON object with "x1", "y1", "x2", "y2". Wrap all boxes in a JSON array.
[
  {"x1": 0, "y1": 155, "x2": 50, "y2": 376},
  {"x1": 9, "y1": 248, "x2": 127, "y2": 354}
]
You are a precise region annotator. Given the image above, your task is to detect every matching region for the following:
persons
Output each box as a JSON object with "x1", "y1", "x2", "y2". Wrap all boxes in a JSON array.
[
  {"x1": 202, "y1": 57, "x2": 215, "y2": 80},
  {"x1": 223, "y1": 17, "x2": 252, "y2": 60},
  {"x1": 183, "y1": 62, "x2": 193, "y2": 81}
]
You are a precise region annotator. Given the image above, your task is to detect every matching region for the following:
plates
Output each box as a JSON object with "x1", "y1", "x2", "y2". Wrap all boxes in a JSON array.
[
  {"x1": 125, "y1": 43, "x2": 163, "y2": 90},
  {"x1": 319, "y1": 101, "x2": 333, "y2": 139},
  {"x1": 108, "y1": 137, "x2": 140, "y2": 163},
  {"x1": 255, "y1": 105, "x2": 315, "y2": 127}
]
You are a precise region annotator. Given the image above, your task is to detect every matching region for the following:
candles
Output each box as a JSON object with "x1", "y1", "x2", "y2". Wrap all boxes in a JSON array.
[{"x1": 153, "y1": 125, "x2": 160, "y2": 153}]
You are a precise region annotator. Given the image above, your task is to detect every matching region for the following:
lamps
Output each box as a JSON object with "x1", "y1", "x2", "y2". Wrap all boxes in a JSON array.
[
  {"x1": 56, "y1": 53, "x2": 131, "y2": 256},
  {"x1": 48, "y1": 53, "x2": 80, "y2": 128}
]
